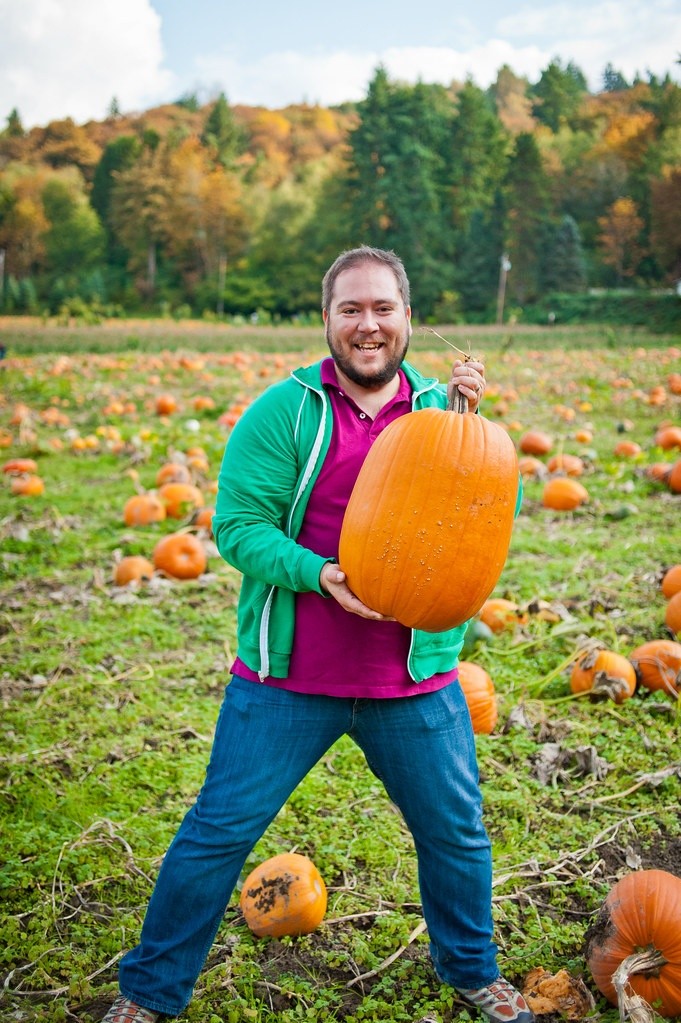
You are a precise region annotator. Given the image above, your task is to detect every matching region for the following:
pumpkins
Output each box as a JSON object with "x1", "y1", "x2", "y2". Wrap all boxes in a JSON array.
[{"x1": 0, "y1": 338, "x2": 681, "y2": 1020}]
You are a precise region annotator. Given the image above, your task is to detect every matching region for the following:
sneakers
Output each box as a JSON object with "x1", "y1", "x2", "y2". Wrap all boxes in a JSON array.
[
  {"x1": 100, "y1": 990, "x2": 161, "y2": 1023},
  {"x1": 435, "y1": 968, "x2": 535, "y2": 1023}
]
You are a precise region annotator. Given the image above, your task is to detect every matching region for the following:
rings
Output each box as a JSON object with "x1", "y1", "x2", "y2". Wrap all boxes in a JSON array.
[{"x1": 474, "y1": 385, "x2": 480, "y2": 392}]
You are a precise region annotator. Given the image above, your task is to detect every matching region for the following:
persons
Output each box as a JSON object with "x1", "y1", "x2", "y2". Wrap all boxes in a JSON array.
[{"x1": 97, "y1": 244, "x2": 537, "y2": 1023}]
